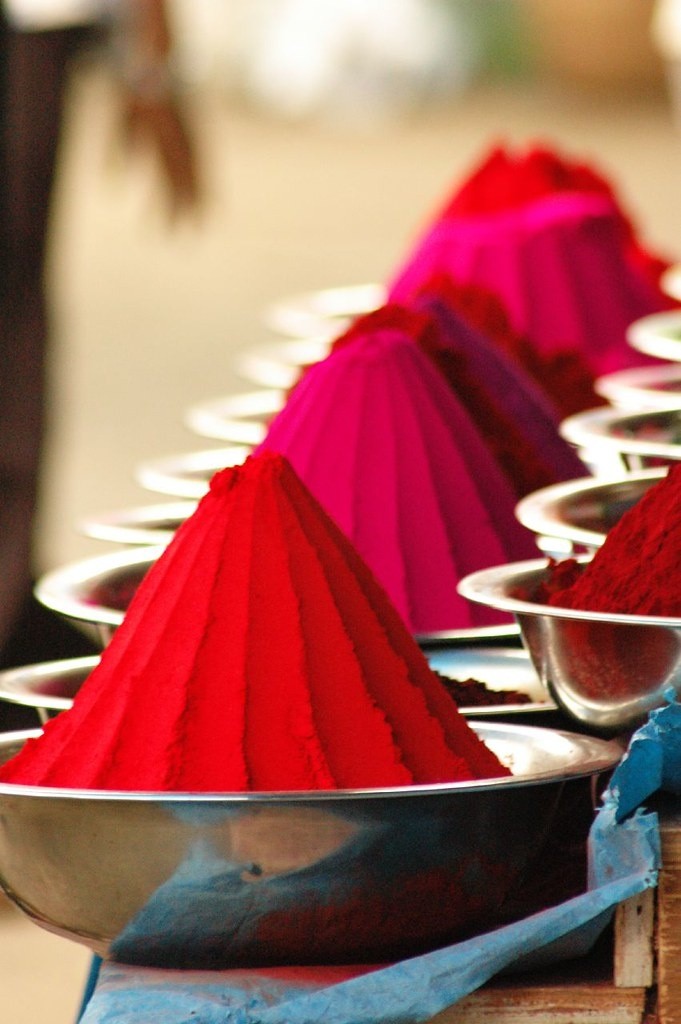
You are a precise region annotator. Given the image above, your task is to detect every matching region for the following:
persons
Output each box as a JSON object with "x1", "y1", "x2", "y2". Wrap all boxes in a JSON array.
[{"x1": 0, "y1": 1, "x2": 202, "y2": 634}]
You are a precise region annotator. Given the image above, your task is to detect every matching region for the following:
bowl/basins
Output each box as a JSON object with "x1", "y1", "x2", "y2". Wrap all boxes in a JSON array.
[{"x1": 0, "y1": 261, "x2": 681, "y2": 976}]
